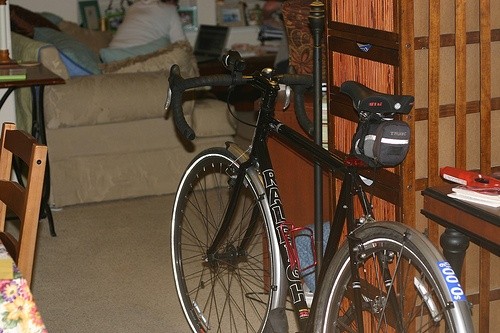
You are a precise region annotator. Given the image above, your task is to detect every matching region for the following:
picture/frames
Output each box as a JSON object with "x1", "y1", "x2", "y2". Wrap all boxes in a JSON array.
[
  {"x1": 217, "y1": 3, "x2": 246, "y2": 27},
  {"x1": 178, "y1": 6, "x2": 199, "y2": 31},
  {"x1": 78, "y1": 1, "x2": 101, "y2": 31}
]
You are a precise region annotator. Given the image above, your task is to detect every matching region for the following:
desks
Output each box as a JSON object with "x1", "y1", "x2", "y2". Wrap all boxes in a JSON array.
[{"x1": 420, "y1": 174, "x2": 500, "y2": 257}]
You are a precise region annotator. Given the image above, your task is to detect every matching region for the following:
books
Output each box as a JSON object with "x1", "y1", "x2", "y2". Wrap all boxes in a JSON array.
[{"x1": 446, "y1": 177, "x2": 500, "y2": 207}]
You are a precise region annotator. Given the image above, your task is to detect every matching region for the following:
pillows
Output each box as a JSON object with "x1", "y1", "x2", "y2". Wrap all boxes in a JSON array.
[
  {"x1": 104, "y1": 40, "x2": 199, "y2": 78},
  {"x1": 34, "y1": 26, "x2": 103, "y2": 77},
  {"x1": 9, "y1": 3, "x2": 62, "y2": 41}
]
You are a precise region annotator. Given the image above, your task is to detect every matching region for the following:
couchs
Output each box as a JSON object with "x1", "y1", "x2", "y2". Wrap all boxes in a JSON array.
[{"x1": 11, "y1": 31, "x2": 241, "y2": 209}]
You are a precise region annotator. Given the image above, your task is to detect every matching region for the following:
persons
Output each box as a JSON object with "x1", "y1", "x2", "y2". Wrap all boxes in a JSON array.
[
  {"x1": 108, "y1": 0, "x2": 187, "y2": 48},
  {"x1": 261, "y1": 0, "x2": 327, "y2": 82}
]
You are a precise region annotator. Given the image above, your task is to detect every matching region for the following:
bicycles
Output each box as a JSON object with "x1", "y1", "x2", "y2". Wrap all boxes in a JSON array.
[{"x1": 162, "y1": 49, "x2": 476, "y2": 333}]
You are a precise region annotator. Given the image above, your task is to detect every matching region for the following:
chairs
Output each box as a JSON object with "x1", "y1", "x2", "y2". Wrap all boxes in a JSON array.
[{"x1": 0, "y1": 122, "x2": 48, "y2": 287}]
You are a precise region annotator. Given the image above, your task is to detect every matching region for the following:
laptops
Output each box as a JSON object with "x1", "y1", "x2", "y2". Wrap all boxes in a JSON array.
[{"x1": 193, "y1": 24, "x2": 230, "y2": 63}]
